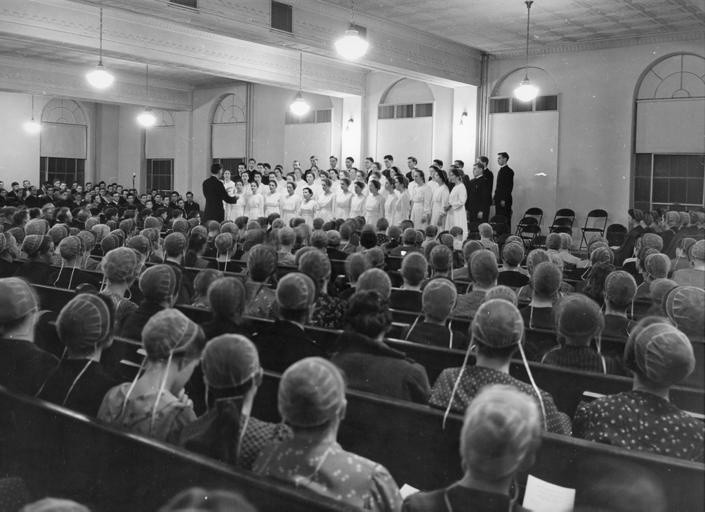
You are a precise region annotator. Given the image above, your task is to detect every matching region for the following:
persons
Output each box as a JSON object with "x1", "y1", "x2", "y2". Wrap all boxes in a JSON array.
[
  {"x1": 2, "y1": 178, "x2": 704, "y2": 511},
  {"x1": 201, "y1": 152, "x2": 514, "y2": 243}
]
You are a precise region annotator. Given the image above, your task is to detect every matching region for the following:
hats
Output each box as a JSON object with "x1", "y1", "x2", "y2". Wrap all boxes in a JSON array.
[
  {"x1": 467, "y1": 298, "x2": 530, "y2": 350},
  {"x1": 556, "y1": 293, "x2": 604, "y2": 334},
  {"x1": 198, "y1": 333, "x2": 265, "y2": 391},
  {"x1": 623, "y1": 313, "x2": 699, "y2": 391},
  {"x1": 54, "y1": 289, "x2": 113, "y2": 351},
  {"x1": 135, "y1": 305, "x2": 204, "y2": 361},
  {"x1": 457, "y1": 384, "x2": 539, "y2": 484},
  {"x1": 0, "y1": 216, "x2": 704, "y2": 317},
  {"x1": 275, "y1": 351, "x2": 350, "y2": 428}
]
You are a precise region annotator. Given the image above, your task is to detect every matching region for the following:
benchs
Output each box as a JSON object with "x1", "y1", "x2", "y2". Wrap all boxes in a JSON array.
[{"x1": 0, "y1": 179, "x2": 705, "y2": 512}]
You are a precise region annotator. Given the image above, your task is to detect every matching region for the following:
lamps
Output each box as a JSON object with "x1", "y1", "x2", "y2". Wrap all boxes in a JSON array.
[
  {"x1": 86, "y1": 5, "x2": 116, "y2": 89},
  {"x1": 21, "y1": 94, "x2": 41, "y2": 133},
  {"x1": 134, "y1": 62, "x2": 157, "y2": 127},
  {"x1": 288, "y1": 52, "x2": 311, "y2": 116},
  {"x1": 513, "y1": 0, "x2": 540, "y2": 104},
  {"x1": 332, "y1": 2, "x2": 371, "y2": 62}
]
